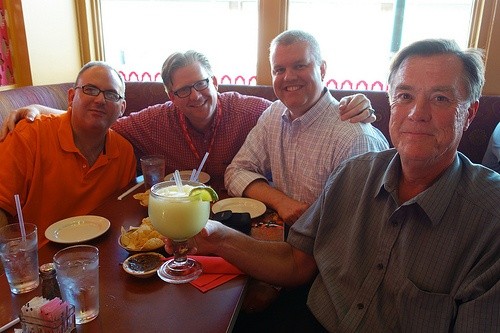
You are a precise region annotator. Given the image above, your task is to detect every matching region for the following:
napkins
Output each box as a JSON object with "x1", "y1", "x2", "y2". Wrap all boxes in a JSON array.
[{"x1": 162, "y1": 254, "x2": 245, "y2": 293}]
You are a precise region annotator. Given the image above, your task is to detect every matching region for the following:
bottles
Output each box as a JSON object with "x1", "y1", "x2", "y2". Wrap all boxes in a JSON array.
[{"x1": 39, "y1": 263, "x2": 62, "y2": 300}]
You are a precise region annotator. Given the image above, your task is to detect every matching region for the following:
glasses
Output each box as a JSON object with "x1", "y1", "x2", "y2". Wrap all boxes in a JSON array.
[
  {"x1": 172, "y1": 75, "x2": 211, "y2": 98},
  {"x1": 74, "y1": 83, "x2": 125, "y2": 102}
]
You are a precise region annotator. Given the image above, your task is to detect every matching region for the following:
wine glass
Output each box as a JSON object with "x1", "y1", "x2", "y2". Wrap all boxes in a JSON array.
[{"x1": 148, "y1": 181, "x2": 210, "y2": 284}]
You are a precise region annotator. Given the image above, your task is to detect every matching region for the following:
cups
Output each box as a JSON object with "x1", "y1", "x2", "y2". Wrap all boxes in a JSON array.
[
  {"x1": 53, "y1": 245, "x2": 99, "y2": 324},
  {"x1": 140, "y1": 155, "x2": 164, "y2": 190},
  {"x1": 0, "y1": 223, "x2": 40, "y2": 294}
]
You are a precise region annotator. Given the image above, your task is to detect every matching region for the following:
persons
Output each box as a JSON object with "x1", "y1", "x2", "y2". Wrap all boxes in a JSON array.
[
  {"x1": 0, "y1": 61, "x2": 136, "y2": 258},
  {"x1": 164, "y1": 40, "x2": 500, "y2": 333},
  {"x1": 0, "y1": 51, "x2": 377, "y2": 195},
  {"x1": 224, "y1": 30, "x2": 390, "y2": 312}
]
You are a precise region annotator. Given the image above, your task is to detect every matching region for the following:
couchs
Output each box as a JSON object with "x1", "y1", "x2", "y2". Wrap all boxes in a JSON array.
[{"x1": 0, "y1": 81, "x2": 500, "y2": 165}]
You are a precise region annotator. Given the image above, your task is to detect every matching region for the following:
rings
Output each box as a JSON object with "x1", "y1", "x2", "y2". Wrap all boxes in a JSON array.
[{"x1": 368, "y1": 107, "x2": 376, "y2": 115}]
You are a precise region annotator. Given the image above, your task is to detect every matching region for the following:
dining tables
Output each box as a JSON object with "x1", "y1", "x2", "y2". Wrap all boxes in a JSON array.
[{"x1": 0, "y1": 175, "x2": 249, "y2": 333}]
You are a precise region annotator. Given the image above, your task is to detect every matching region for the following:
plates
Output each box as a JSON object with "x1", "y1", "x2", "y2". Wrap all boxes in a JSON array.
[
  {"x1": 44, "y1": 216, "x2": 110, "y2": 243},
  {"x1": 164, "y1": 170, "x2": 210, "y2": 183},
  {"x1": 123, "y1": 253, "x2": 165, "y2": 278},
  {"x1": 212, "y1": 197, "x2": 266, "y2": 218},
  {"x1": 119, "y1": 229, "x2": 164, "y2": 252}
]
